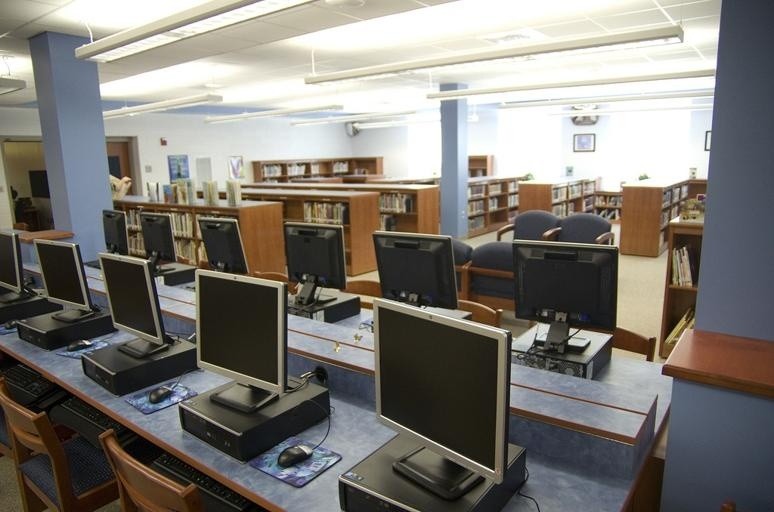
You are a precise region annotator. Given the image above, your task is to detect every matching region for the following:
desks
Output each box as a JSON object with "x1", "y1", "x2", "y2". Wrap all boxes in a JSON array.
[
  {"x1": 1, "y1": 267, "x2": 647, "y2": 511},
  {"x1": 80, "y1": 262, "x2": 675, "y2": 443}
]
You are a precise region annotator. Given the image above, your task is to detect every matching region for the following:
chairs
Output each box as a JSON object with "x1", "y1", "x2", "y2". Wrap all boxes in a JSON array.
[
  {"x1": 470, "y1": 241, "x2": 519, "y2": 312},
  {"x1": 496, "y1": 208, "x2": 562, "y2": 242},
  {"x1": 176, "y1": 255, "x2": 190, "y2": 265},
  {"x1": 458, "y1": 298, "x2": 503, "y2": 330},
  {"x1": 448, "y1": 237, "x2": 473, "y2": 301},
  {"x1": 99, "y1": 429, "x2": 200, "y2": 512},
  {"x1": 338, "y1": 278, "x2": 383, "y2": 308},
  {"x1": 253, "y1": 270, "x2": 298, "y2": 294},
  {"x1": 550, "y1": 212, "x2": 614, "y2": 246},
  {"x1": 0, "y1": 392, "x2": 120, "y2": 512},
  {"x1": 199, "y1": 260, "x2": 214, "y2": 271}
]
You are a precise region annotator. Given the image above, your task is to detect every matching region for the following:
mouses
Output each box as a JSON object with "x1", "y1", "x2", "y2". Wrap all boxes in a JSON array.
[
  {"x1": 67, "y1": 338, "x2": 93, "y2": 351},
  {"x1": 5, "y1": 319, "x2": 20, "y2": 329},
  {"x1": 150, "y1": 386, "x2": 173, "y2": 404},
  {"x1": 278, "y1": 444, "x2": 314, "y2": 467}
]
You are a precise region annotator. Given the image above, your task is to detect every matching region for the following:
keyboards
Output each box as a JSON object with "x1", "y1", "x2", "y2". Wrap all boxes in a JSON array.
[
  {"x1": 153, "y1": 451, "x2": 251, "y2": 511},
  {"x1": 61, "y1": 396, "x2": 127, "y2": 434},
  {"x1": 3, "y1": 364, "x2": 56, "y2": 398}
]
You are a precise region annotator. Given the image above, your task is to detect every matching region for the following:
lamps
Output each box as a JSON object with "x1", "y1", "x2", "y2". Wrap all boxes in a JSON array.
[
  {"x1": 74, "y1": 1, "x2": 302, "y2": 66},
  {"x1": 202, "y1": 103, "x2": 344, "y2": 124},
  {"x1": 0, "y1": 47, "x2": 26, "y2": 95},
  {"x1": 305, "y1": 2, "x2": 684, "y2": 86},
  {"x1": 102, "y1": 77, "x2": 225, "y2": 119},
  {"x1": 282, "y1": 108, "x2": 417, "y2": 127},
  {"x1": 426, "y1": 69, "x2": 716, "y2": 100}
]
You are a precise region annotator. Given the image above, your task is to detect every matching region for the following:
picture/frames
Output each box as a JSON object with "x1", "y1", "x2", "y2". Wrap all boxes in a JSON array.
[
  {"x1": 228, "y1": 156, "x2": 245, "y2": 178},
  {"x1": 168, "y1": 154, "x2": 191, "y2": 183},
  {"x1": 705, "y1": 129, "x2": 712, "y2": 150},
  {"x1": 573, "y1": 132, "x2": 596, "y2": 152}
]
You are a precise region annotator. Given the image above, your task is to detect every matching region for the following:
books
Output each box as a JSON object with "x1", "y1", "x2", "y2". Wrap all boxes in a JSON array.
[
  {"x1": 672, "y1": 246, "x2": 698, "y2": 288},
  {"x1": 552, "y1": 182, "x2": 596, "y2": 219},
  {"x1": 123, "y1": 180, "x2": 243, "y2": 263},
  {"x1": 467, "y1": 180, "x2": 527, "y2": 230},
  {"x1": 303, "y1": 202, "x2": 349, "y2": 226},
  {"x1": 380, "y1": 193, "x2": 414, "y2": 232},
  {"x1": 665, "y1": 308, "x2": 695, "y2": 346},
  {"x1": 595, "y1": 197, "x2": 623, "y2": 221},
  {"x1": 261, "y1": 162, "x2": 348, "y2": 184},
  {"x1": 659, "y1": 186, "x2": 688, "y2": 250}
]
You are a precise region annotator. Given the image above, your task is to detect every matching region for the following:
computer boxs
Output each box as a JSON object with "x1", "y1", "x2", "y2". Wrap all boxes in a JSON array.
[
  {"x1": 0, "y1": 288, "x2": 63, "y2": 324},
  {"x1": 155, "y1": 262, "x2": 200, "y2": 286},
  {"x1": 287, "y1": 291, "x2": 361, "y2": 324},
  {"x1": 81, "y1": 333, "x2": 199, "y2": 396},
  {"x1": 511, "y1": 322, "x2": 614, "y2": 380},
  {"x1": 338, "y1": 434, "x2": 527, "y2": 512},
  {"x1": 17, "y1": 306, "x2": 118, "y2": 350},
  {"x1": 421, "y1": 305, "x2": 473, "y2": 320},
  {"x1": 178, "y1": 375, "x2": 330, "y2": 462}
]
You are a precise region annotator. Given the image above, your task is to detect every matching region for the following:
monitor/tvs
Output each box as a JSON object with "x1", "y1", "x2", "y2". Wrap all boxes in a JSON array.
[
  {"x1": 96, "y1": 252, "x2": 174, "y2": 358},
  {"x1": 197, "y1": 217, "x2": 249, "y2": 275},
  {"x1": 140, "y1": 211, "x2": 178, "y2": 274},
  {"x1": 373, "y1": 297, "x2": 512, "y2": 502},
  {"x1": 283, "y1": 221, "x2": 347, "y2": 306},
  {"x1": 0, "y1": 231, "x2": 38, "y2": 304},
  {"x1": 33, "y1": 239, "x2": 96, "y2": 323},
  {"x1": 194, "y1": 268, "x2": 289, "y2": 414},
  {"x1": 371, "y1": 230, "x2": 459, "y2": 310},
  {"x1": 102, "y1": 209, "x2": 129, "y2": 255},
  {"x1": 512, "y1": 239, "x2": 619, "y2": 353}
]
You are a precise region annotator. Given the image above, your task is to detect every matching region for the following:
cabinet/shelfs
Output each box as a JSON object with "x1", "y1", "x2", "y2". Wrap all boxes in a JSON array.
[
  {"x1": 621, "y1": 179, "x2": 708, "y2": 257},
  {"x1": 241, "y1": 186, "x2": 381, "y2": 277},
  {"x1": 467, "y1": 176, "x2": 525, "y2": 238},
  {"x1": 469, "y1": 155, "x2": 493, "y2": 178},
  {"x1": 115, "y1": 195, "x2": 285, "y2": 277},
  {"x1": 240, "y1": 182, "x2": 440, "y2": 236},
  {"x1": 594, "y1": 192, "x2": 624, "y2": 224},
  {"x1": 517, "y1": 179, "x2": 596, "y2": 219},
  {"x1": 252, "y1": 155, "x2": 384, "y2": 185},
  {"x1": 660, "y1": 213, "x2": 704, "y2": 358}
]
